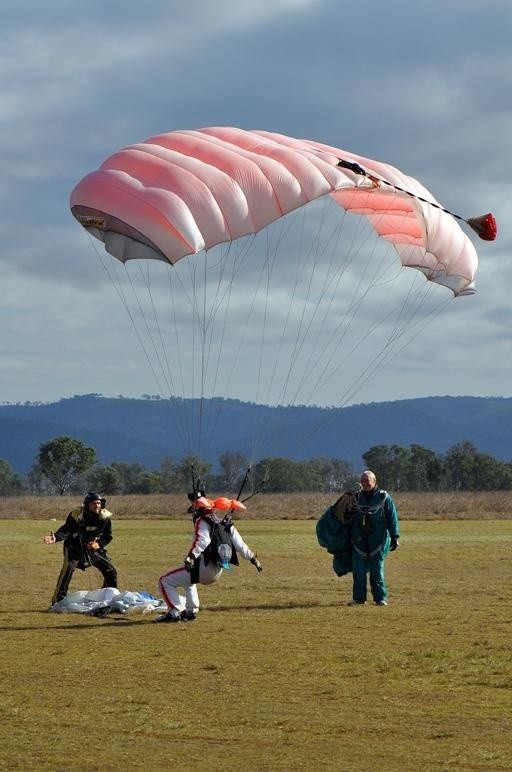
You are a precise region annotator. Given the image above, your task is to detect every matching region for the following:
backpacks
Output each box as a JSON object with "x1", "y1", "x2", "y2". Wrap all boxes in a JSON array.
[{"x1": 193, "y1": 513, "x2": 238, "y2": 567}]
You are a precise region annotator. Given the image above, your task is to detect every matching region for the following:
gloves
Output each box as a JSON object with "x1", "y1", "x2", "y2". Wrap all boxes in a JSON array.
[
  {"x1": 184, "y1": 554, "x2": 195, "y2": 572},
  {"x1": 250, "y1": 555, "x2": 263, "y2": 572}
]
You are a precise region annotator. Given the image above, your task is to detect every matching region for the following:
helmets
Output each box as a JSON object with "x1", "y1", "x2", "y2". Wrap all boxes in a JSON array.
[{"x1": 84, "y1": 492, "x2": 106, "y2": 510}]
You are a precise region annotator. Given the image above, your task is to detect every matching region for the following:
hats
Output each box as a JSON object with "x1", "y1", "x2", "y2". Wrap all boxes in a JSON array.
[{"x1": 187, "y1": 489, "x2": 206, "y2": 502}]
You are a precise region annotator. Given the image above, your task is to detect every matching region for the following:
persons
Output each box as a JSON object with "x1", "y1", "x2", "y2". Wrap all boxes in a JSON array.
[
  {"x1": 45, "y1": 492, "x2": 118, "y2": 610},
  {"x1": 315, "y1": 470, "x2": 400, "y2": 607},
  {"x1": 160, "y1": 497, "x2": 264, "y2": 622}
]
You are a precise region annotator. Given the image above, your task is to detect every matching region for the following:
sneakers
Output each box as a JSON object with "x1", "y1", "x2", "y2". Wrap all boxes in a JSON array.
[
  {"x1": 348, "y1": 599, "x2": 388, "y2": 606},
  {"x1": 154, "y1": 609, "x2": 197, "y2": 622}
]
